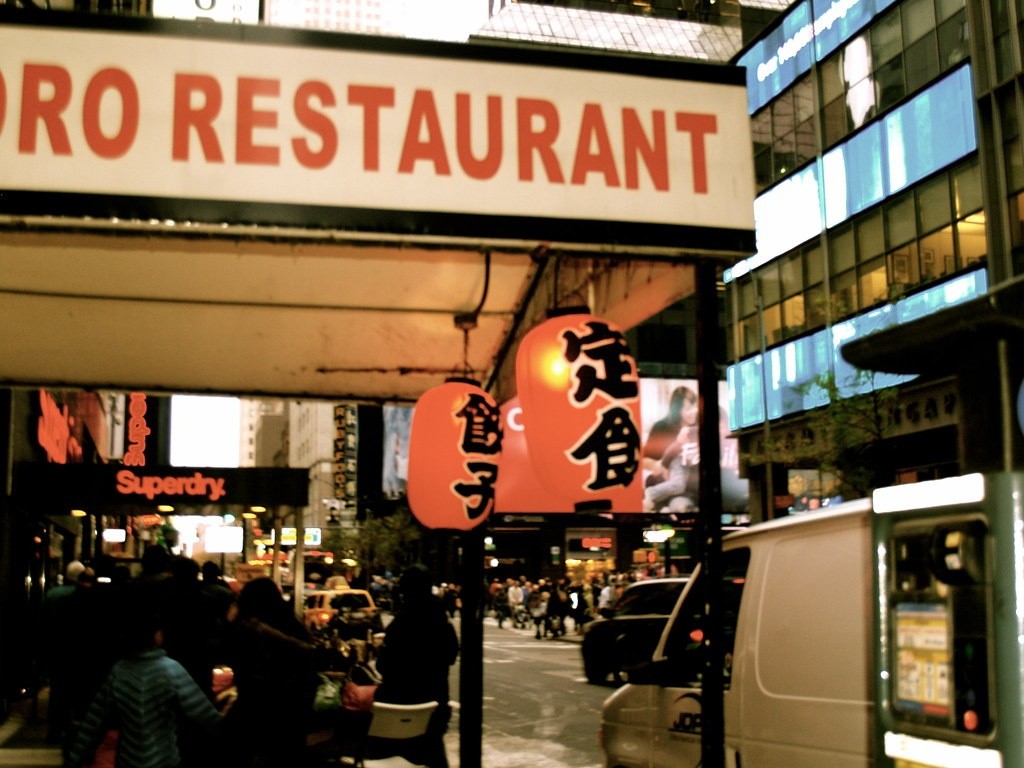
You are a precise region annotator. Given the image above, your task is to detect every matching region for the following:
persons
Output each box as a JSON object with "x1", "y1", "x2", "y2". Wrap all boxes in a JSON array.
[
  {"x1": 343, "y1": 566, "x2": 460, "y2": 768},
  {"x1": 23, "y1": 546, "x2": 348, "y2": 767},
  {"x1": 640, "y1": 385, "x2": 697, "y2": 512},
  {"x1": 371, "y1": 564, "x2": 680, "y2": 640},
  {"x1": 661, "y1": 400, "x2": 751, "y2": 514}
]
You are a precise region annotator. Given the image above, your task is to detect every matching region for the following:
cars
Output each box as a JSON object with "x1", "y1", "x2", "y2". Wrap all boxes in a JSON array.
[
  {"x1": 578, "y1": 578, "x2": 688, "y2": 684},
  {"x1": 305, "y1": 589, "x2": 376, "y2": 624}
]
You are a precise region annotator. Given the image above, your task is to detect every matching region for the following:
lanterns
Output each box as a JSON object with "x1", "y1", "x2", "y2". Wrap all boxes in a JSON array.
[
  {"x1": 515, "y1": 304, "x2": 645, "y2": 515},
  {"x1": 406, "y1": 375, "x2": 503, "y2": 539}
]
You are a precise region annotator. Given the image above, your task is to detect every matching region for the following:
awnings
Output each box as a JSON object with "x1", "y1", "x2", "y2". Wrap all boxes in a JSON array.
[{"x1": 841, "y1": 274, "x2": 1024, "y2": 378}]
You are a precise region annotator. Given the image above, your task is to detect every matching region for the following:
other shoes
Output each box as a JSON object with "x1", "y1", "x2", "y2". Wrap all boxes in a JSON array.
[{"x1": 497, "y1": 624, "x2": 584, "y2": 640}]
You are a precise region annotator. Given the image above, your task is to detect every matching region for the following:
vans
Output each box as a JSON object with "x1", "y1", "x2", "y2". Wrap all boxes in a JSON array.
[{"x1": 597, "y1": 498, "x2": 880, "y2": 767}]
